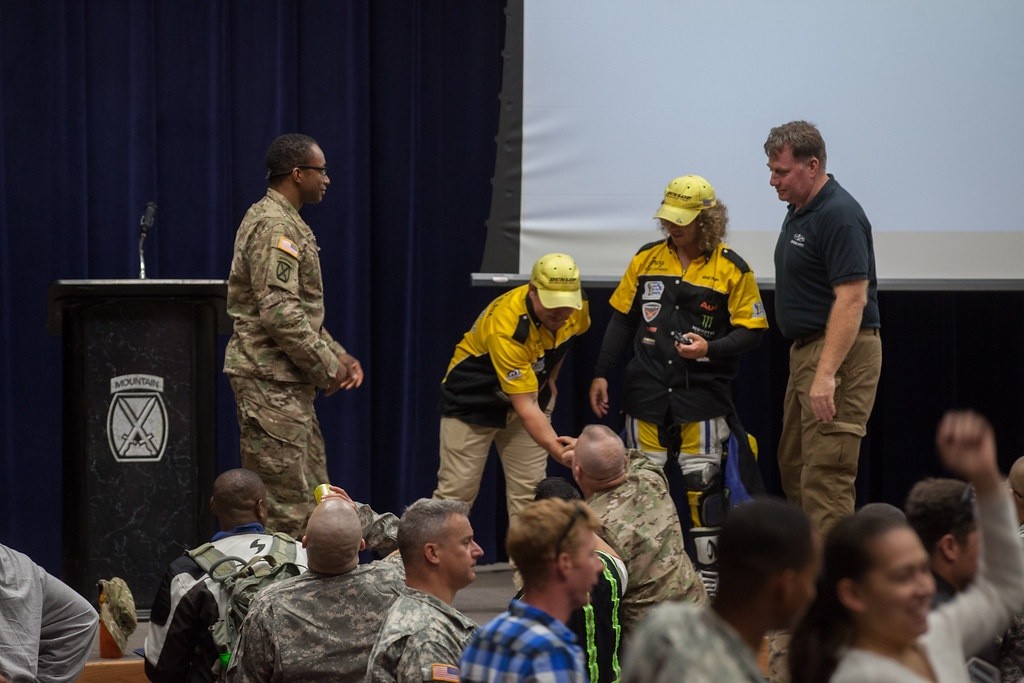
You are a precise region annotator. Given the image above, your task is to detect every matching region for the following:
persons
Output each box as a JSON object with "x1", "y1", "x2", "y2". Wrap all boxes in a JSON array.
[
  {"x1": 590, "y1": 174, "x2": 769, "y2": 601},
  {"x1": 0, "y1": 546, "x2": 100, "y2": 683},
  {"x1": 764, "y1": 122, "x2": 883, "y2": 561},
  {"x1": 132, "y1": 411, "x2": 1024, "y2": 683},
  {"x1": 431, "y1": 254, "x2": 591, "y2": 600},
  {"x1": 221, "y1": 134, "x2": 365, "y2": 543}
]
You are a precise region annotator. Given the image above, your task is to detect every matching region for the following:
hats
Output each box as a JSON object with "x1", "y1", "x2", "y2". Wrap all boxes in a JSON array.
[
  {"x1": 98, "y1": 577, "x2": 137, "y2": 656},
  {"x1": 530, "y1": 252, "x2": 583, "y2": 311},
  {"x1": 652, "y1": 174, "x2": 716, "y2": 227}
]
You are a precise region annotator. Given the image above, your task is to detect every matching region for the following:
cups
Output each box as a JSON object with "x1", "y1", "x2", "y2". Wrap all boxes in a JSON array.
[{"x1": 314, "y1": 484, "x2": 336, "y2": 506}]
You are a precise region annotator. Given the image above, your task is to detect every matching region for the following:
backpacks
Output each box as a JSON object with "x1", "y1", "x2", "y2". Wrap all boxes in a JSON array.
[{"x1": 184, "y1": 532, "x2": 300, "y2": 683}]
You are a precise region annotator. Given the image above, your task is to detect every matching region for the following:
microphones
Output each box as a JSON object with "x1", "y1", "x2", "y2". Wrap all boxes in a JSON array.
[{"x1": 141, "y1": 202, "x2": 158, "y2": 234}]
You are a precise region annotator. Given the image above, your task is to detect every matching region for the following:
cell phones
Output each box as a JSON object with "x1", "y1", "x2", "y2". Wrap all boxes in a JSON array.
[{"x1": 670, "y1": 331, "x2": 691, "y2": 344}]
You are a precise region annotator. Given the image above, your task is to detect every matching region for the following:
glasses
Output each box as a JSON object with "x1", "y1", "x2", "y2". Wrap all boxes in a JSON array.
[{"x1": 298, "y1": 165, "x2": 329, "y2": 176}]
